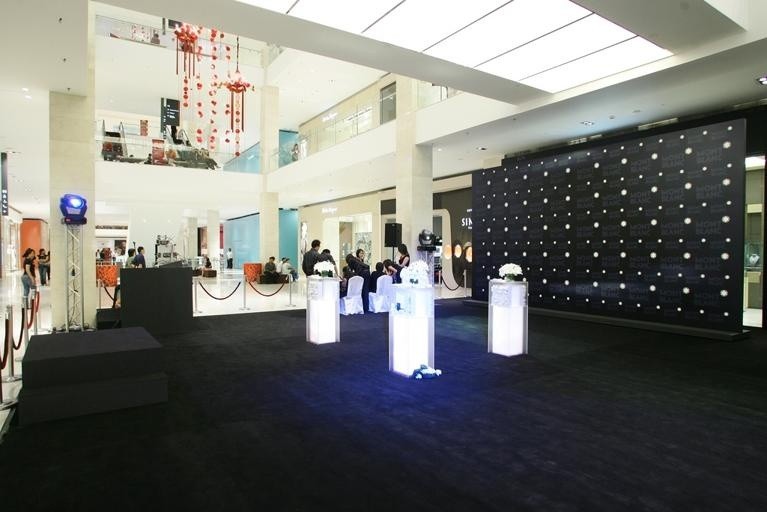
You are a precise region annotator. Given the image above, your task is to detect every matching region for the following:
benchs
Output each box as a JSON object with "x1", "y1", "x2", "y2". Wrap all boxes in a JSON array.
[{"x1": 258, "y1": 272, "x2": 289, "y2": 284}]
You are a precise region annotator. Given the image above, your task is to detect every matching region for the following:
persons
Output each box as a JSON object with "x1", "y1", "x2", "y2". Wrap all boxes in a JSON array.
[
  {"x1": 143, "y1": 153, "x2": 151, "y2": 165},
  {"x1": 302, "y1": 238, "x2": 412, "y2": 315},
  {"x1": 226, "y1": 247, "x2": 234, "y2": 269},
  {"x1": 262, "y1": 256, "x2": 278, "y2": 284},
  {"x1": 94, "y1": 247, "x2": 116, "y2": 265},
  {"x1": 20, "y1": 246, "x2": 37, "y2": 309},
  {"x1": 36, "y1": 248, "x2": 49, "y2": 286},
  {"x1": 289, "y1": 144, "x2": 300, "y2": 162},
  {"x1": 151, "y1": 33, "x2": 160, "y2": 45},
  {"x1": 281, "y1": 258, "x2": 299, "y2": 282},
  {"x1": 124, "y1": 248, "x2": 136, "y2": 267},
  {"x1": 203, "y1": 257, "x2": 212, "y2": 268},
  {"x1": 275, "y1": 256, "x2": 286, "y2": 274},
  {"x1": 130, "y1": 246, "x2": 146, "y2": 268}
]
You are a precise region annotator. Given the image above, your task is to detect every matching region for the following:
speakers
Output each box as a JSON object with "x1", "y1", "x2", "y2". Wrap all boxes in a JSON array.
[{"x1": 385, "y1": 223, "x2": 402, "y2": 247}]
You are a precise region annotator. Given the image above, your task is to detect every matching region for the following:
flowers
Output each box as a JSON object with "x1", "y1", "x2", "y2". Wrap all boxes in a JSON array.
[
  {"x1": 313, "y1": 259, "x2": 335, "y2": 277},
  {"x1": 399, "y1": 259, "x2": 430, "y2": 285},
  {"x1": 498, "y1": 261, "x2": 524, "y2": 282}
]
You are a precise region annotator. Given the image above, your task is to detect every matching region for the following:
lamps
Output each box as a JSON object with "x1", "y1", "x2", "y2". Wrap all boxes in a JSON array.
[{"x1": 59, "y1": 193, "x2": 89, "y2": 227}]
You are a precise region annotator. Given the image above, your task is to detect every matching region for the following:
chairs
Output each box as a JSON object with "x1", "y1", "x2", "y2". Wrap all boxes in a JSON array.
[
  {"x1": 368, "y1": 275, "x2": 393, "y2": 314},
  {"x1": 340, "y1": 275, "x2": 364, "y2": 315}
]
